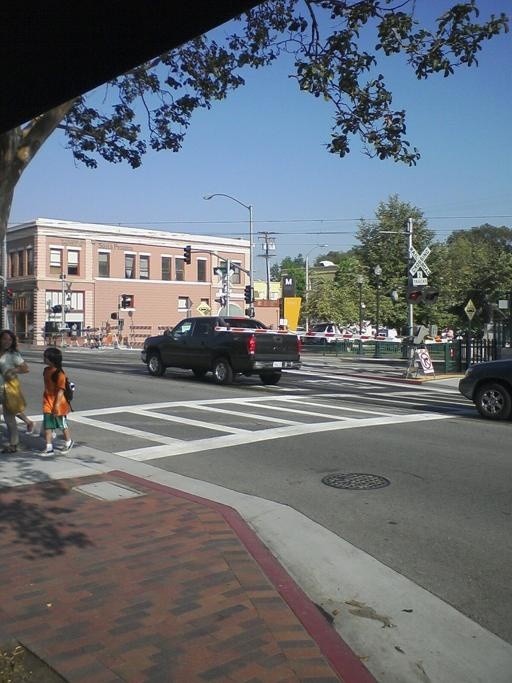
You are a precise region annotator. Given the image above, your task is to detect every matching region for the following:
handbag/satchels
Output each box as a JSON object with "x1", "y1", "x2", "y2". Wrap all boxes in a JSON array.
[{"x1": 4, "y1": 377, "x2": 27, "y2": 413}]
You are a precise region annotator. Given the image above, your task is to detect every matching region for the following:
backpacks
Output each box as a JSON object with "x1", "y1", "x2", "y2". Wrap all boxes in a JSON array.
[{"x1": 41, "y1": 367, "x2": 74, "y2": 403}]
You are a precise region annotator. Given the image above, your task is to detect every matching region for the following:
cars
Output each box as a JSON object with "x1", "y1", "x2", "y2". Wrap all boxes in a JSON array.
[
  {"x1": 458, "y1": 359, "x2": 512, "y2": 419},
  {"x1": 304, "y1": 322, "x2": 352, "y2": 343}
]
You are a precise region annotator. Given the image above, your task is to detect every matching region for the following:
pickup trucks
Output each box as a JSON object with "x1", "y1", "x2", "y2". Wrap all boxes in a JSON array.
[{"x1": 141, "y1": 316, "x2": 301, "y2": 385}]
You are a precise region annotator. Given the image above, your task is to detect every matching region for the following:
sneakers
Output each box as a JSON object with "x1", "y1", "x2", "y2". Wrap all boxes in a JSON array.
[
  {"x1": 60, "y1": 439, "x2": 74, "y2": 454},
  {"x1": 25, "y1": 421, "x2": 35, "y2": 435},
  {"x1": 39, "y1": 450, "x2": 55, "y2": 456},
  {"x1": 3, "y1": 445, "x2": 16, "y2": 453}
]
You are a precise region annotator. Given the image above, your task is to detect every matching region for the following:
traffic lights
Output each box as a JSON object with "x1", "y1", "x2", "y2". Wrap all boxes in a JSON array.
[
  {"x1": 5, "y1": 286, "x2": 14, "y2": 305},
  {"x1": 182, "y1": 245, "x2": 192, "y2": 264},
  {"x1": 122, "y1": 294, "x2": 130, "y2": 309},
  {"x1": 407, "y1": 288, "x2": 439, "y2": 305},
  {"x1": 244, "y1": 285, "x2": 252, "y2": 303}
]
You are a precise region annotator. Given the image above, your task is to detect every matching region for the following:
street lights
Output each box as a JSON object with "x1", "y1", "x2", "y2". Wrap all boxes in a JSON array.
[
  {"x1": 373, "y1": 265, "x2": 383, "y2": 358},
  {"x1": 203, "y1": 193, "x2": 253, "y2": 318},
  {"x1": 303, "y1": 243, "x2": 329, "y2": 332},
  {"x1": 357, "y1": 273, "x2": 365, "y2": 354}
]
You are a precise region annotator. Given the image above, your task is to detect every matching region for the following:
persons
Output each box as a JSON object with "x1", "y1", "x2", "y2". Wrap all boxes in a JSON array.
[
  {"x1": 0, "y1": 329, "x2": 34, "y2": 453},
  {"x1": 39, "y1": 347, "x2": 75, "y2": 456}
]
